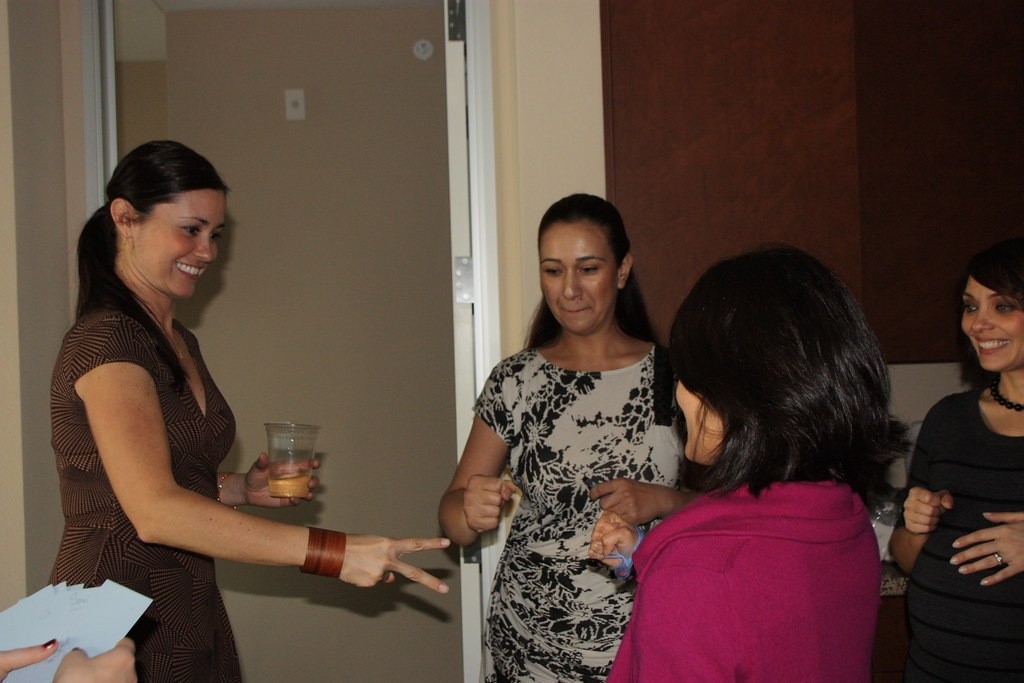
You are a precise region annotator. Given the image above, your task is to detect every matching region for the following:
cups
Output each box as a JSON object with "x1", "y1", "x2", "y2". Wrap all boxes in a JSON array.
[{"x1": 265, "y1": 422, "x2": 321, "y2": 497}]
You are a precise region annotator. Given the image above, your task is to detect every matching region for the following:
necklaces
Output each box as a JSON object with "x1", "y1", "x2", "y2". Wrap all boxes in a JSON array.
[{"x1": 990, "y1": 375, "x2": 1024, "y2": 412}]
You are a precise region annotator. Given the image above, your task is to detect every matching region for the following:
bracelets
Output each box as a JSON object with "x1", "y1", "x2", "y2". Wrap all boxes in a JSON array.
[
  {"x1": 217, "y1": 472, "x2": 237, "y2": 509},
  {"x1": 606, "y1": 526, "x2": 645, "y2": 580},
  {"x1": 299, "y1": 527, "x2": 347, "y2": 578}
]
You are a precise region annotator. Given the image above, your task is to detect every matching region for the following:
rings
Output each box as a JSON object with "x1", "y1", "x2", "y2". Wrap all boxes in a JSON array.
[{"x1": 994, "y1": 553, "x2": 1003, "y2": 566}]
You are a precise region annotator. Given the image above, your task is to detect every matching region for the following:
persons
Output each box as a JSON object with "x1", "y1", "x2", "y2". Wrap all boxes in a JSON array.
[
  {"x1": 587, "y1": 249, "x2": 913, "y2": 683},
  {"x1": 48, "y1": 140, "x2": 450, "y2": 683},
  {"x1": 437, "y1": 193, "x2": 685, "y2": 683},
  {"x1": 0, "y1": 637, "x2": 138, "y2": 683},
  {"x1": 888, "y1": 239, "x2": 1024, "y2": 683}
]
